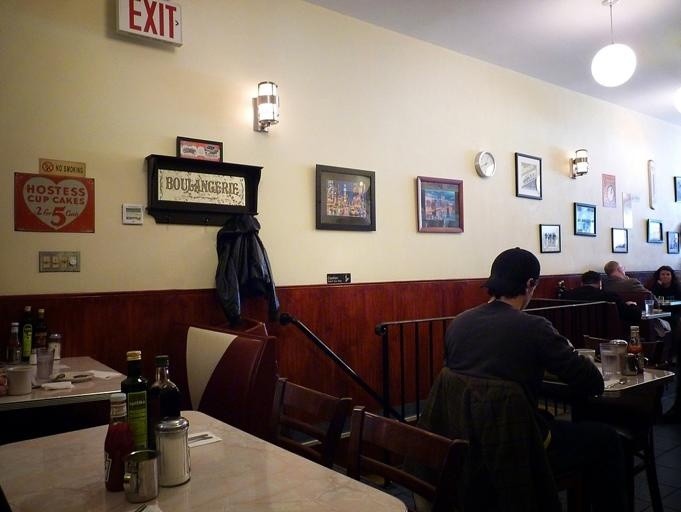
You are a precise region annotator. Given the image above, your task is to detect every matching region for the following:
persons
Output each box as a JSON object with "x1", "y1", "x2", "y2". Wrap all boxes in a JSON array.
[
  {"x1": 600, "y1": 262, "x2": 672, "y2": 364},
  {"x1": 565, "y1": 268, "x2": 642, "y2": 325},
  {"x1": 445, "y1": 245, "x2": 627, "y2": 510},
  {"x1": 647, "y1": 266, "x2": 680, "y2": 301}
]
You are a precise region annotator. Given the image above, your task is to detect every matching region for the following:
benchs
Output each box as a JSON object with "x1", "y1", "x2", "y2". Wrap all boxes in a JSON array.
[{"x1": 146, "y1": 319, "x2": 280, "y2": 445}]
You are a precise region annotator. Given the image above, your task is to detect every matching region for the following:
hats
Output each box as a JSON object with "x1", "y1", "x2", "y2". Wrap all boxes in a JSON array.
[{"x1": 480, "y1": 246, "x2": 543, "y2": 290}]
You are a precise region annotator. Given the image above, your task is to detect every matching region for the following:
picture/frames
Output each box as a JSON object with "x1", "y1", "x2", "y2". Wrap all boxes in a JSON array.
[
  {"x1": 514, "y1": 152, "x2": 544, "y2": 201},
  {"x1": 573, "y1": 201, "x2": 598, "y2": 237},
  {"x1": 176, "y1": 135, "x2": 223, "y2": 164},
  {"x1": 610, "y1": 226, "x2": 630, "y2": 254},
  {"x1": 674, "y1": 176, "x2": 681, "y2": 203},
  {"x1": 417, "y1": 174, "x2": 467, "y2": 236},
  {"x1": 646, "y1": 218, "x2": 666, "y2": 244},
  {"x1": 666, "y1": 230, "x2": 681, "y2": 255},
  {"x1": 314, "y1": 163, "x2": 378, "y2": 234},
  {"x1": 539, "y1": 223, "x2": 563, "y2": 254}
]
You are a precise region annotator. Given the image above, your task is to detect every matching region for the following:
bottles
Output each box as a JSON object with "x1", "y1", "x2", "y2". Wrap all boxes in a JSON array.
[
  {"x1": 103, "y1": 393, "x2": 137, "y2": 492},
  {"x1": 7, "y1": 305, "x2": 63, "y2": 363},
  {"x1": 121, "y1": 350, "x2": 150, "y2": 456},
  {"x1": 149, "y1": 354, "x2": 181, "y2": 456},
  {"x1": 611, "y1": 325, "x2": 645, "y2": 375},
  {"x1": 155, "y1": 418, "x2": 190, "y2": 485}
]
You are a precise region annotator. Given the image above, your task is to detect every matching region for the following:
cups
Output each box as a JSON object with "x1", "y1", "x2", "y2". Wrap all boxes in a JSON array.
[
  {"x1": 645, "y1": 299, "x2": 655, "y2": 315},
  {"x1": 121, "y1": 450, "x2": 160, "y2": 501},
  {"x1": 577, "y1": 348, "x2": 596, "y2": 359},
  {"x1": 1, "y1": 365, "x2": 33, "y2": 395},
  {"x1": 600, "y1": 343, "x2": 627, "y2": 387},
  {"x1": 659, "y1": 296, "x2": 665, "y2": 303},
  {"x1": 36, "y1": 347, "x2": 55, "y2": 379}
]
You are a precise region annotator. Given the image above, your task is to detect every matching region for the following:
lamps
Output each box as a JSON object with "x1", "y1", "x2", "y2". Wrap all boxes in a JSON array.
[
  {"x1": 590, "y1": 0, "x2": 640, "y2": 91},
  {"x1": 253, "y1": 80, "x2": 283, "y2": 136},
  {"x1": 568, "y1": 148, "x2": 591, "y2": 181}
]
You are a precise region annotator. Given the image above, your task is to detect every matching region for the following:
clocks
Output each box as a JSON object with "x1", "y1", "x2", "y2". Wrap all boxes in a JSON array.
[{"x1": 474, "y1": 150, "x2": 497, "y2": 179}]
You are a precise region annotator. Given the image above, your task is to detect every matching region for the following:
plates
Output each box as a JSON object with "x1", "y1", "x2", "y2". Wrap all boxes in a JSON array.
[{"x1": 50, "y1": 371, "x2": 94, "y2": 382}]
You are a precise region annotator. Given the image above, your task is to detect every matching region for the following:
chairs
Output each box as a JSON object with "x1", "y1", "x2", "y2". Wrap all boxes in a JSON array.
[
  {"x1": 413, "y1": 364, "x2": 563, "y2": 511},
  {"x1": 270, "y1": 376, "x2": 355, "y2": 469},
  {"x1": 345, "y1": 404, "x2": 472, "y2": 511}
]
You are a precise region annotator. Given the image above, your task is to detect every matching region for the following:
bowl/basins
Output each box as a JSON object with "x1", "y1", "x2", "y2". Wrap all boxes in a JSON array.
[{"x1": 654, "y1": 309, "x2": 663, "y2": 314}]
